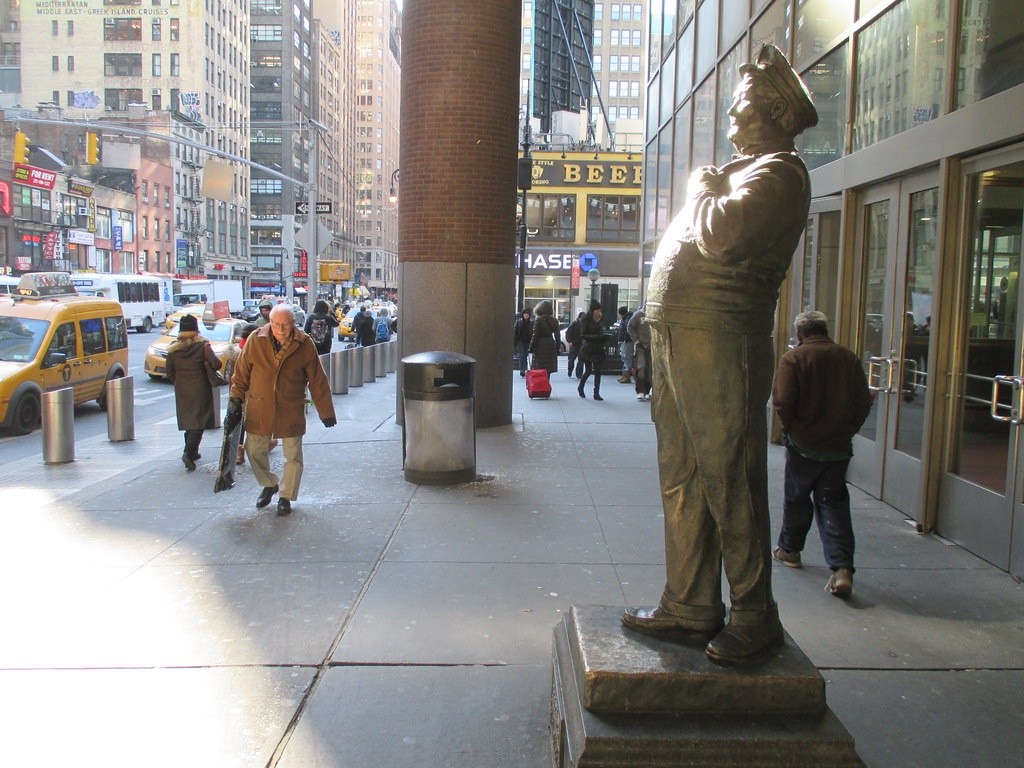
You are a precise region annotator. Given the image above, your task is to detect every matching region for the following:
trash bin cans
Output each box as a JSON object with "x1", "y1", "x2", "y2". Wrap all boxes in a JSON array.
[{"x1": 402, "y1": 352, "x2": 475, "y2": 485}]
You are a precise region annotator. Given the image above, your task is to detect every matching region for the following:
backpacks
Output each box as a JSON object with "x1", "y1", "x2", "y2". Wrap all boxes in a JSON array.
[{"x1": 375, "y1": 317, "x2": 390, "y2": 341}]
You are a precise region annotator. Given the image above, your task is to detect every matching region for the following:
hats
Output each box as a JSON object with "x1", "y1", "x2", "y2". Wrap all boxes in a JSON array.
[
  {"x1": 590, "y1": 299, "x2": 601, "y2": 311},
  {"x1": 178, "y1": 314, "x2": 199, "y2": 333},
  {"x1": 619, "y1": 306, "x2": 628, "y2": 315},
  {"x1": 578, "y1": 312, "x2": 586, "y2": 318}
]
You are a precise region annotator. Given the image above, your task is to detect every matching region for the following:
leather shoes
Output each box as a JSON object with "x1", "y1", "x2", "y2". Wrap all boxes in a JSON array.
[
  {"x1": 276, "y1": 497, "x2": 291, "y2": 515},
  {"x1": 256, "y1": 484, "x2": 279, "y2": 508}
]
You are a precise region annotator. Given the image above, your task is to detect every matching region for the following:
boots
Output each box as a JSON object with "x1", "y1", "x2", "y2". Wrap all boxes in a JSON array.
[
  {"x1": 577, "y1": 382, "x2": 586, "y2": 398},
  {"x1": 593, "y1": 389, "x2": 603, "y2": 400}
]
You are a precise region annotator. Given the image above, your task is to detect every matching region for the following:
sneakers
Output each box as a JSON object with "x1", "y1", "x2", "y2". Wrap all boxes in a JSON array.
[
  {"x1": 772, "y1": 546, "x2": 803, "y2": 567},
  {"x1": 824, "y1": 569, "x2": 854, "y2": 597}
]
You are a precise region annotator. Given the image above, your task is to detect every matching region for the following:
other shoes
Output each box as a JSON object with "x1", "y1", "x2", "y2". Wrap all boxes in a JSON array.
[
  {"x1": 182, "y1": 452, "x2": 201, "y2": 471},
  {"x1": 617, "y1": 373, "x2": 631, "y2": 383},
  {"x1": 637, "y1": 393, "x2": 650, "y2": 401}
]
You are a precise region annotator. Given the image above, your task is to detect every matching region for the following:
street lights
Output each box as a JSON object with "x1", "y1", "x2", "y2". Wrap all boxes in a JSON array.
[
  {"x1": 280, "y1": 247, "x2": 288, "y2": 295},
  {"x1": 512, "y1": 77, "x2": 537, "y2": 369},
  {"x1": 588, "y1": 269, "x2": 600, "y2": 299},
  {"x1": 547, "y1": 276, "x2": 554, "y2": 314}
]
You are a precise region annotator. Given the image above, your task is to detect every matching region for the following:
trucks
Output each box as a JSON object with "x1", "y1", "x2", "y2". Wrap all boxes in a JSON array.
[
  {"x1": 243, "y1": 299, "x2": 262, "y2": 307},
  {"x1": 168, "y1": 279, "x2": 243, "y2": 312}
]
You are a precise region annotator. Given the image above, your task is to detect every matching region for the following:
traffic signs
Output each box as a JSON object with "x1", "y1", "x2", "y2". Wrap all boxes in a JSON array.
[{"x1": 295, "y1": 201, "x2": 332, "y2": 214}]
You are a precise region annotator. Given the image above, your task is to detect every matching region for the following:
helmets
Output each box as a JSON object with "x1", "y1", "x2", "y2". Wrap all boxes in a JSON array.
[{"x1": 258, "y1": 299, "x2": 273, "y2": 311}]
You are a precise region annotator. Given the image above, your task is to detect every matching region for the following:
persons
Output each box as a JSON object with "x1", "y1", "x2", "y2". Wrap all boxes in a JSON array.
[
  {"x1": 772, "y1": 311, "x2": 871, "y2": 595},
  {"x1": 621, "y1": 43, "x2": 818, "y2": 665},
  {"x1": 234, "y1": 295, "x2": 398, "y2": 464},
  {"x1": 165, "y1": 315, "x2": 222, "y2": 468},
  {"x1": 229, "y1": 304, "x2": 337, "y2": 515},
  {"x1": 866, "y1": 312, "x2": 931, "y2": 401},
  {"x1": 514, "y1": 299, "x2": 653, "y2": 400}
]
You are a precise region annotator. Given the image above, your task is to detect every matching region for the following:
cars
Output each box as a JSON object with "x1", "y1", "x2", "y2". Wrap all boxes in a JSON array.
[
  {"x1": 144, "y1": 315, "x2": 249, "y2": 384},
  {"x1": 292, "y1": 304, "x2": 305, "y2": 327},
  {"x1": 335, "y1": 299, "x2": 397, "y2": 342},
  {"x1": 241, "y1": 305, "x2": 262, "y2": 322},
  {"x1": 552, "y1": 328, "x2": 569, "y2": 356},
  {"x1": 166, "y1": 303, "x2": 207, "y2": 329}
]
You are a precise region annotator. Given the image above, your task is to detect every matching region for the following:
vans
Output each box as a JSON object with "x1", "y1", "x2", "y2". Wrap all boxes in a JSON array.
[{"x1": 0, "y1": 294, "x2": 128, "y2": 436}]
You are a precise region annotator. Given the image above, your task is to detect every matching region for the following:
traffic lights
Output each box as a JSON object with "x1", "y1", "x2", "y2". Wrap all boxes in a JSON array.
[
  {"x1": 13, "y1": 133, "x2": 31, "y2": 164},
  {"x1": 88, "y1": 134, "x2": 99, "y2": 165}
]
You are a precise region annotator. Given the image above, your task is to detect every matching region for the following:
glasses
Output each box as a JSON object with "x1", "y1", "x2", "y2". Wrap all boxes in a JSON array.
[
  {"x1": 270, "y1": 319, "x2": 294, "y2": 329},
  {"x1": 524, "y1": 312, "x2": 529, "y2": 314}
]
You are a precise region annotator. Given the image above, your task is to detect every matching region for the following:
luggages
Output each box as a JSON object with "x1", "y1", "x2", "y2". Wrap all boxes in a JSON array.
[{"x1": 524, "y1": 350, "x2": 552, "y2": 400}]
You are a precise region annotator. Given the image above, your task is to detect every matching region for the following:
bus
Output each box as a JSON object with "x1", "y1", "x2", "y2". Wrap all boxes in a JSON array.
[{"x1": 70, "y1": 274, "x2": 166, "y2": 333}]
